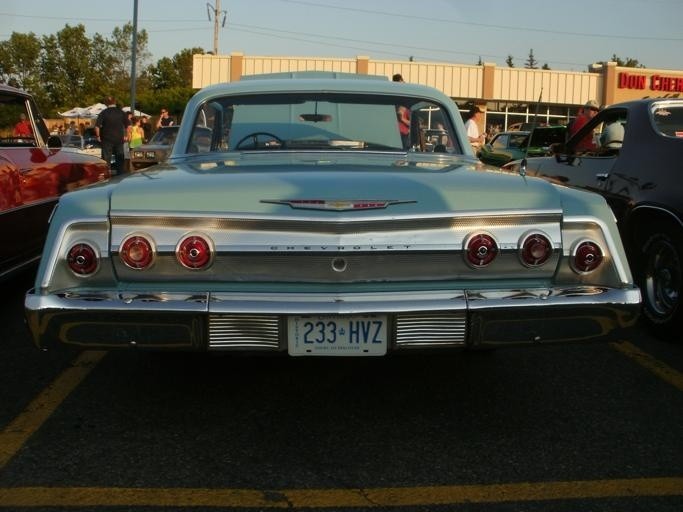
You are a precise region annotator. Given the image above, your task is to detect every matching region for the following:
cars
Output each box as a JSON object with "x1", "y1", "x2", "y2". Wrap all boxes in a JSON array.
[
  {"x1": 0, "y1": 81, "x2": 112, "y2": 295},
  {"x1": 500, "y1": 96, "x2": 683, "y2": 337},
  {"x1": 21, "y1": 69, "x2": 644, "y2": 359}
]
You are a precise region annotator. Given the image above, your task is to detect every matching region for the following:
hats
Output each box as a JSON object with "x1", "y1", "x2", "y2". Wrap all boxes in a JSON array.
[
  {"x1": 584, "y1": 101, "x2": 599, "y2": 111},
  {"x1": 105, "y1": 96, "x2": 115, "y2": 104},
  {"x1": 473, "y1": 104, "x2": 486, "y2": 113}
]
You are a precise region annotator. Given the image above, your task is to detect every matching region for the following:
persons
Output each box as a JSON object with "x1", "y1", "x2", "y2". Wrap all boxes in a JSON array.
[
  {"x1": 13, "y1": 95, "x2": 228, "y2": 174},
  {"x1": 393, "y1": 99, "x2": 625, "y2": 162}
]
